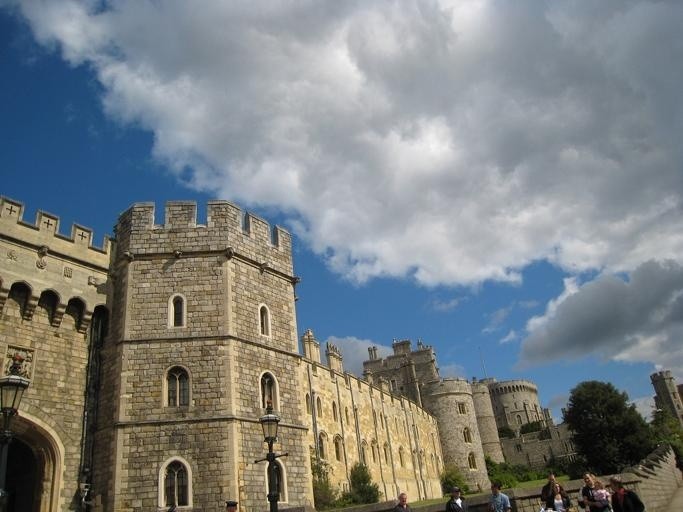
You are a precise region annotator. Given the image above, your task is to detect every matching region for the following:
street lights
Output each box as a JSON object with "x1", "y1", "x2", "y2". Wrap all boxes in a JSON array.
[
  {"x1": 253, "y1": 399, "x2": 287, "y2": 511},
  {"x1": 0, "y1": 351, "x2": 31, "y2": 512}
]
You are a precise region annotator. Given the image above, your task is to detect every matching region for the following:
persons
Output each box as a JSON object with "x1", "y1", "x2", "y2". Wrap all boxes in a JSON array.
[
  {"x1": 446, "y1": 487, "x2": 468, "y2": 512},
  {"x1": 395, "y1": 493, "x2": 412, "y2": 512},
  {"x1": 545, "y1": 481, "x2": 569, "y2": 512},
  {"x1": 541, "y1": 473, "x2": 566, "y2": 501},
  {"x1": 576, "y1": 470, "x2": 644, "y2": 512},
  {"x1": 487, "y1": 482, "x2": 512, "y2": 512}
]
